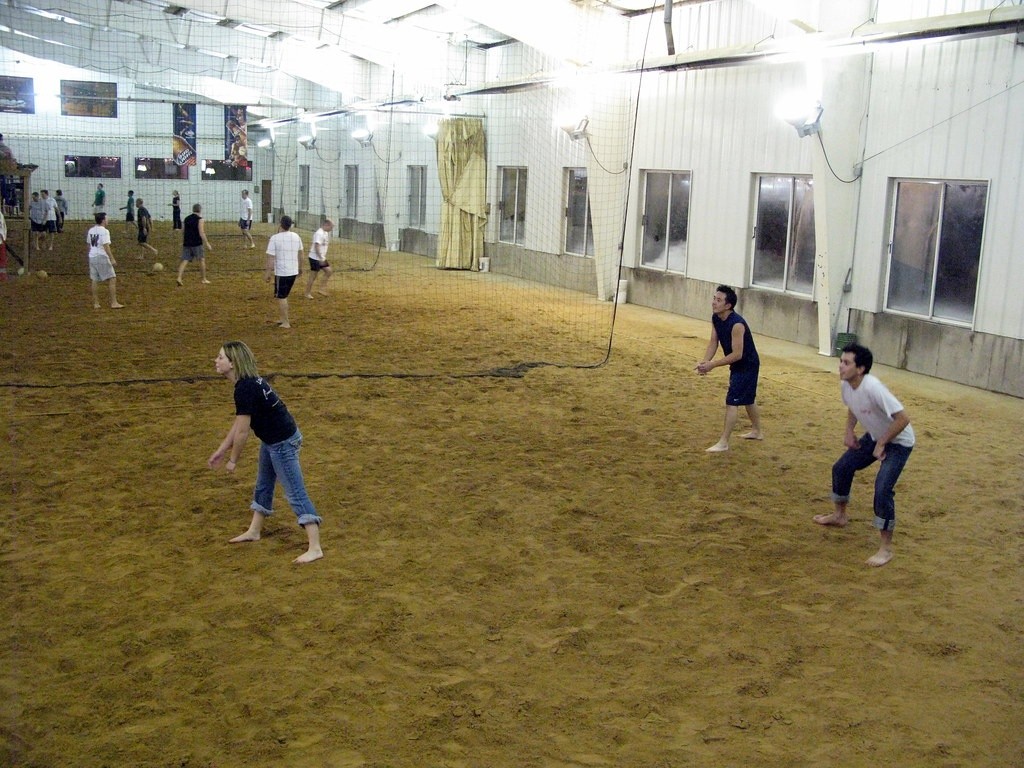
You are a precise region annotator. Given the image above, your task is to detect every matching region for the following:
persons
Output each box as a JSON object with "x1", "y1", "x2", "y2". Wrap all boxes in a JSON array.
[
  {"x1": 53, "y1": 189, "x2": 68, "y2": 233},
  {"x1": 134, "y1": 198, "x2": 158, "y2": 259},
  {"x1": 87, "y1": 212, "x2": 124, "y2": 309},
  {"x1": 168, "y1": 190, "x2": 182, "y2": 231},
  {"x1": 237, "y1": 189, "x2": 255, "y2": 249},
  {"x1": 265, "y1": 216, "x2": 304, "y2": 329},
  {"x1": 0, "y1": 211, "x2": 8, "y2": 281},
  {"x1": 225, "y1": 107, "x2": 248, "y2": 168},
  {"x1": 119, "y1": 190, "x2": 138, "y2": 234},
  {"x1": 813, "y1": 342, "x2": 915, "y2": 566},
  {"x1": 176, "y1": 204, "x2": 212, "y2": 286},
  {"x1": 305, "y1": 220, "x2": 334, "y2": 299},
  {"x1": 28, "y1": 191, "x2": 49, "y2": 250},
  {"x1": 693, "y1": 285, "x2": 764, "y2": 452},
  {"x1": 178, "y1": 104, "x2": 195, "y2": 146},
  {"x1": 208, "y1": 341, "x2": 324, "y2": 564},
  {"x1": 40, "y1": 190, "x2": 62, "y2": 250},
  {"x1": 92, "y1": 183, "x2": 106, "y2": 215}
]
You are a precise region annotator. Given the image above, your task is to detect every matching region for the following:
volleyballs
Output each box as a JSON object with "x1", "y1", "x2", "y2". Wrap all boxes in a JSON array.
[
  {"x1": 37, "y1": 270, "x2": 48, "y2": 280},
  {"x1": 17, "y1": 267, "x2": 25, "y2": 274},
  {"x1": 153, "y1": 262, "x2": 164, "y2": 272}
]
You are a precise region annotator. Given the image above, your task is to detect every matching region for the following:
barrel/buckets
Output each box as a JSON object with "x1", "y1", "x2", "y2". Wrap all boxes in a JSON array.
[
  {"x1": 392, "y1": 239, "x2": 400, "y2": 251},
  {"x1": 268, "y1": 213, "x2": 273, "y2": 223},
  {"x1": 613, "y1": 280, "x2": 627, "y2": 304},
  {"x1": 479, "y1": 256, "x2": 490, "y2": 273}
]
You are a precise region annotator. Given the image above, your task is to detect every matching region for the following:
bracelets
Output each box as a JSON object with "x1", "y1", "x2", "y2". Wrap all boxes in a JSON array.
[{"x1": 229, "y1": 459, "x2": 236, "y2": 463}]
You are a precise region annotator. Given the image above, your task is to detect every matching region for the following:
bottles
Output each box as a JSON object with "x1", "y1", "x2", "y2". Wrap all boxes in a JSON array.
[
  {"x1": 227, "y1": 121, "x2": 247, "y2": 148},
  {"x1": 172, "y1": 134, "x2": 196, "y2": 167}
]
